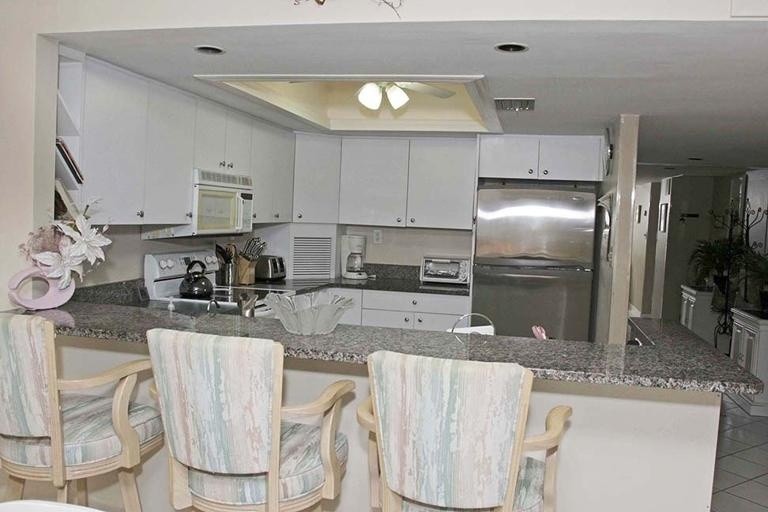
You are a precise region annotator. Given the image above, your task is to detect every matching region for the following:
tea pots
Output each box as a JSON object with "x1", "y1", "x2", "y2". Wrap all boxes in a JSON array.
[{"x1": 180, "y1": 260, "x2": 213, "y2": 298}]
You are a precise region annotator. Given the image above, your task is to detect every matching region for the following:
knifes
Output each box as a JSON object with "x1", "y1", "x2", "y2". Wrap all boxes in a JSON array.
[{"x1": 242, "y1": 236, "x2": 266, "y2": 259}]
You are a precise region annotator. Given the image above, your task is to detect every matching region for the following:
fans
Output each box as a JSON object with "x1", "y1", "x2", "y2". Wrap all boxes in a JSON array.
[{"x1": 287, "y1": 80, "x2": 456, "y2": 99}]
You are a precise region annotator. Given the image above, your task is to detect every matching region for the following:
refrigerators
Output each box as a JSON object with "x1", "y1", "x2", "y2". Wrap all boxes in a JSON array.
[{"x1": 470, "y1": 184, "x2": 599, "y2": 345}]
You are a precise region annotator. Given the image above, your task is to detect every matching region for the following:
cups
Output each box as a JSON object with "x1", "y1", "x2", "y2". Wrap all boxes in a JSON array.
[{"x1": 215, "y1": 262, "x2": 238, "y2": 285}]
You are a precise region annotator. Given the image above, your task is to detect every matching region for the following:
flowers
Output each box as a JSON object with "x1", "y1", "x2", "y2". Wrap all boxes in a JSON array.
[{"x1": 18, "y1": 214, "x2": 113, "y2": 290}]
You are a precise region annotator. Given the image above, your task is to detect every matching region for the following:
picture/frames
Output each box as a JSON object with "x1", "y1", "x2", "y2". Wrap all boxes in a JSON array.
[{"x1": 659, "y1": 203, "x2": 667, "y2": 233}]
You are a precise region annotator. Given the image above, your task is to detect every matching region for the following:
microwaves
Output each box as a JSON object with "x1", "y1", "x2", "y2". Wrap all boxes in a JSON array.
[
  {"x1": 420, "y1": 256, "x2": 470, "y2": 285},
  {"x1": 141, "y1": 188, "x2": 255, "y2": 242}
]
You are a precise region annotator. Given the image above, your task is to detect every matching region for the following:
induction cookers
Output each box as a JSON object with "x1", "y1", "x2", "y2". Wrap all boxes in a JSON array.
[{"x1": 167, "y1": 289, "x2": 297, "y2": 303}]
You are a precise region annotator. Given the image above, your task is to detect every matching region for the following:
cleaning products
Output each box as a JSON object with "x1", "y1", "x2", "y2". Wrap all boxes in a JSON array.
[{"x1": 167, "y1": 296, "x2": 176, "y2": 311}]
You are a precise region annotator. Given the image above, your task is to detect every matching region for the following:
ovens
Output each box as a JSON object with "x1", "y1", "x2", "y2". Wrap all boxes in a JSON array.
[{"x1": 251, "y1": 305, "x2": 277, "y2": 320}]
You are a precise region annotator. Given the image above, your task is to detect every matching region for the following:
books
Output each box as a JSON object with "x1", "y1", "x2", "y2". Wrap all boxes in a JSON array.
[{"x1": 55, "y1": 136, "x2": 85, "y2": 235}]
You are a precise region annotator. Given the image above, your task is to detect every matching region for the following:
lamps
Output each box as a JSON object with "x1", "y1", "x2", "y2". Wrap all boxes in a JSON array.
[
  {"x1": 706, "y1": 198, "x2": 768, "y2": 302},
  {"x1": 357, "y1": 83, "x2": 409, "y2": 110}
]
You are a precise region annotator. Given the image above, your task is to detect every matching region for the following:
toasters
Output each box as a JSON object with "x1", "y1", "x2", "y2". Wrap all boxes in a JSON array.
[{"x1": 255, "y1": 254, "x2": 287, "y2": 281}]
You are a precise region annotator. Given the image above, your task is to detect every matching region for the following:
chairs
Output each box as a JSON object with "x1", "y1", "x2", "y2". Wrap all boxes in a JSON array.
[
  {"x1": 147, "y1": 329, "x2": 356, "y2": 512},
  {"x1": 356, "y1": 350, "x2": 572, "y2": 512},
  {"x1": 0, "y1": 312, "x2": 165, "y2": 512}
]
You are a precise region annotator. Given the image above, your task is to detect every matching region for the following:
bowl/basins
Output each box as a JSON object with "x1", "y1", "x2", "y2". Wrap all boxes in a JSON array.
[{"x1": 265, "y1": 291, "x2": 356, "y2": 336}]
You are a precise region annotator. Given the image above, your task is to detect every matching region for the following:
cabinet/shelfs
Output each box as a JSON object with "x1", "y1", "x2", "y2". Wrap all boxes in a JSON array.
[
  {"x1": 681, "y1": 290, "x2": 732, "y2": 356},
  {"x1": 729, "y1": 315, "x2": 768, "y2": 416},
  {"x1": 56, "y1": 51, "x2": 293, "y2": 226},
  {"x1": 362, "y1": 289, "x2": 469, "y2": 333},
  {"x1": 340, "y1": 135, "x2": 477, "y2": 230},
  {"x1": 292, "y1": 131, "x2": 341, "y2": 224},
  {"x1": 479, "y1": 133, "x2": 603, "y2": 181},
  {"x1": 327, "y1": 287, "x2": 362, "y2": 327}
]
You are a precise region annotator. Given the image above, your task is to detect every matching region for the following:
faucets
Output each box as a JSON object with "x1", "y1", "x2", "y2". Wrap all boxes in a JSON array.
[{"x1": 206, "y1": 299, "x2": 221, "y2": 312}]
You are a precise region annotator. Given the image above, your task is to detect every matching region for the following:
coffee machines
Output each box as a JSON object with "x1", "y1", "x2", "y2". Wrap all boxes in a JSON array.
[{"x1": 340, "y1": 234, "x2": 369, "y2": 280}]
